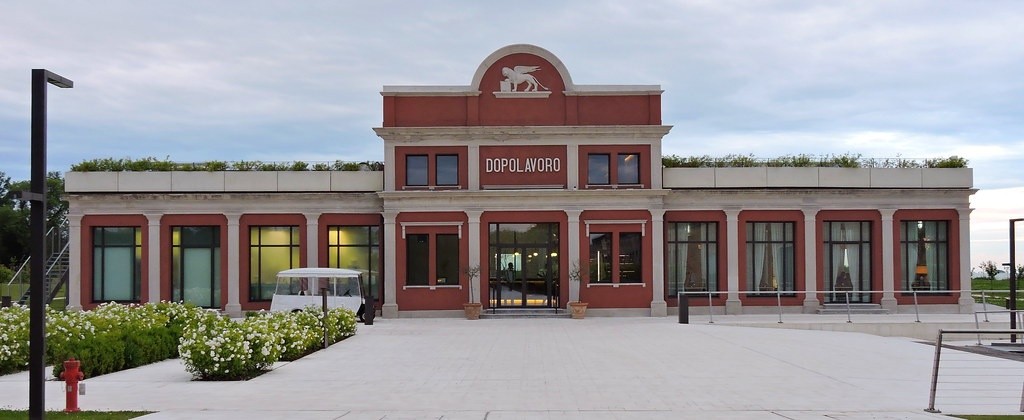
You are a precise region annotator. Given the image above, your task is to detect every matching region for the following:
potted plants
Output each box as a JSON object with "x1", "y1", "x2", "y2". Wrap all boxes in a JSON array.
[
  {"x1": 463, "y1": 263, "x2": 481, "y2": 320},
  {"x1": 569, "y1": 260, "x2": 588, "y2": 318}
]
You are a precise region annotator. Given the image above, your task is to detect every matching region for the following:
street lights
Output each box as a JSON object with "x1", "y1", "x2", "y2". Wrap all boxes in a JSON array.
[
  {"x1": 28, "y1": 68, "x2": 74, "y2": 420},
  {"x1": 1009, "y1": 218, "x2": 1024, "y2": 343}
]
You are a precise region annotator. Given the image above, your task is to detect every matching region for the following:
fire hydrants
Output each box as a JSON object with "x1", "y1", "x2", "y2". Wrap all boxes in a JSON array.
[{"x1": 59, "y1": 356, "x2": 85, "y2": 413}]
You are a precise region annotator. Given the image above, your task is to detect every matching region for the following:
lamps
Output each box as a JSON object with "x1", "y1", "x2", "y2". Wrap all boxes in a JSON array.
[
  {"x1": 533, "y1": 241, "x2": 539, "y2": 256},
  {"x1": 514, "y1": 248, "x2": 519, "y2": 256}
]
